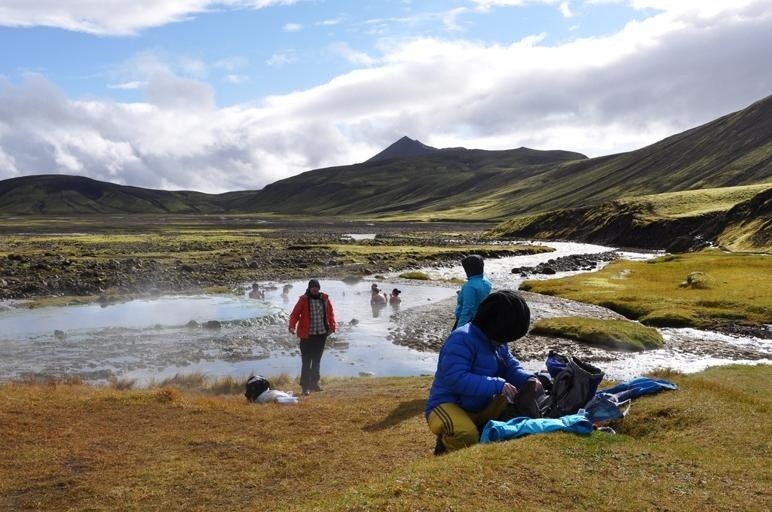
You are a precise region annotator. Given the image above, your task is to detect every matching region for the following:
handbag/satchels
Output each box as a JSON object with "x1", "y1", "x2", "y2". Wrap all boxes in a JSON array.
[
  {"x1": 480, "y1": 350, "x2": 679, "y2": 442},
  {"x1": 245, "y1": 375, "x2": 270, "y2": 402}
]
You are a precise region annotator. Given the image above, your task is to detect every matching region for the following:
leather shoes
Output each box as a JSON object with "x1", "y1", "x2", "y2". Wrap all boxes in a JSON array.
[
  {"x1": 313, "y1": 383, "x2": 321, "y2": 391},
  {"x1": 302, "y1": 387, "x2": 311, "y2": 395}
]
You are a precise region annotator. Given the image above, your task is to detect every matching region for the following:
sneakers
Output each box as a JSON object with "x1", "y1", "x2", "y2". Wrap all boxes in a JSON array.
[{"x1": 435, "y1": 434, "x2": 445, "y2": 455}]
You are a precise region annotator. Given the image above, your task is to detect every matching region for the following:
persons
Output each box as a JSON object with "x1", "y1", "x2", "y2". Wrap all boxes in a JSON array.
[
  {"x1": 371, "y1": 284, "x2": 387, "y2": 305},
  {"x1": 390, "y1": 288, "x2": 401, "y2": 303},
  {"x1": 286, "y1": 278, "x2": 335, "y2": 394},
  {"x1": 450, "y1": 254, "x2": 492, "y2": 331},
  {"x1": 281, "y1": 285, "x2": 289, "y2": 296},
  {"x1": 426, "y1": 290, "x2": 544, "y2": 453},
  {"x1": 248, "y1": 283, "x2": 264, "y2": 299}
]
